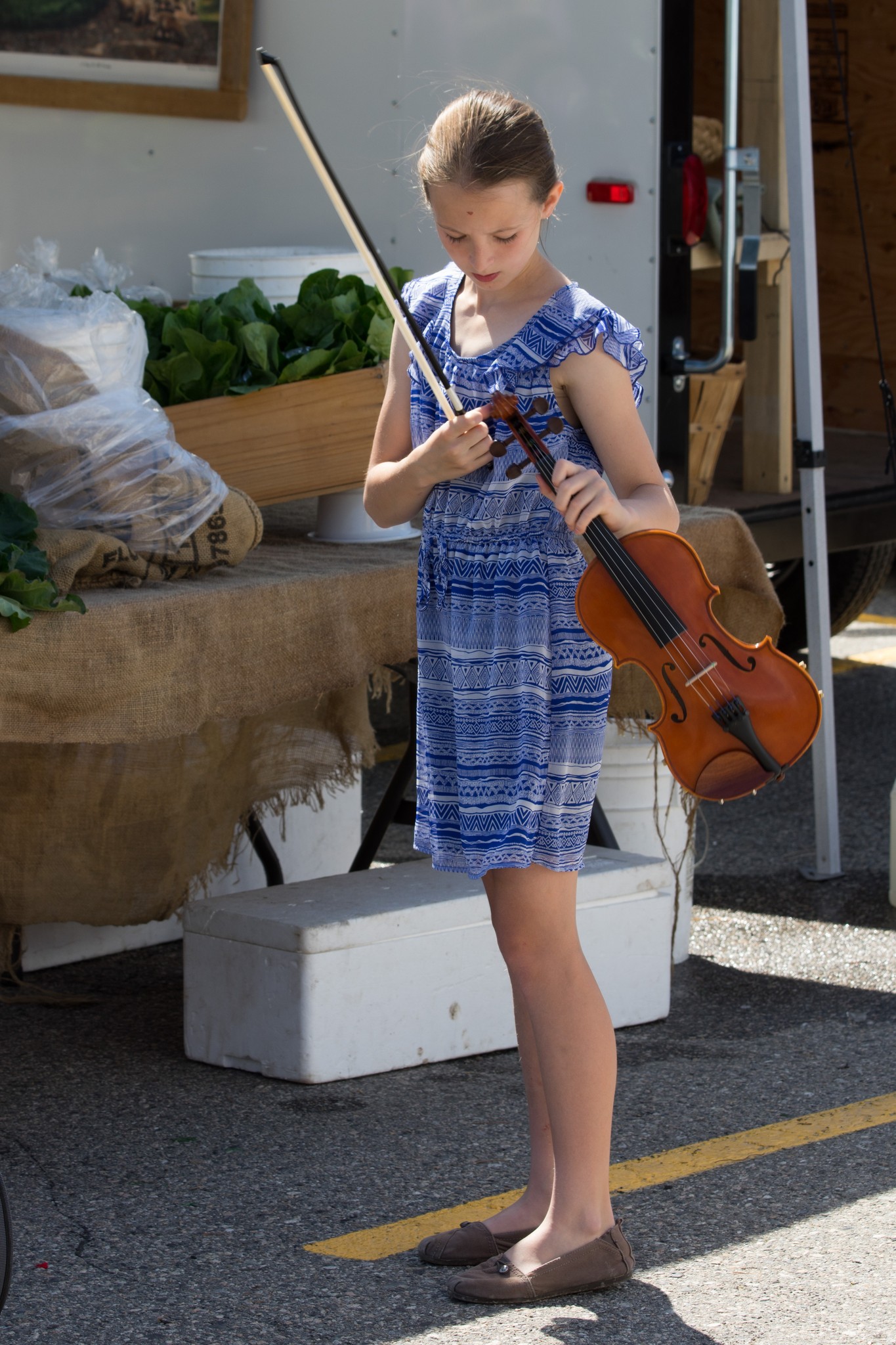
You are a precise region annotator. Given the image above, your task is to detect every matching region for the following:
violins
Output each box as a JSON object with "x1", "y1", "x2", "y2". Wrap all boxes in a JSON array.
[{"x1": 487, "y1": 388, "x2": 826, "y2": 804}]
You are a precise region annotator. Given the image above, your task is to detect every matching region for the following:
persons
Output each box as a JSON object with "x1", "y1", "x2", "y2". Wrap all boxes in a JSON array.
[{"x1": 361, "y1": 91, "x2": 680, "y2": 1305}]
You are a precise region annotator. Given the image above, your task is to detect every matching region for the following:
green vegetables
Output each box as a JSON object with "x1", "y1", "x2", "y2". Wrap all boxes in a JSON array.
[
  {"x1": 71, "y1": 261, "x2": 414, "y2": 407},
  {"x1": 0, "y1": 486, "x2": 88, "y2": 633}
]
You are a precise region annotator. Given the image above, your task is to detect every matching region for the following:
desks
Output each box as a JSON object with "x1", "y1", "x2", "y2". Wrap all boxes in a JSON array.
[{"x1": 0, "y1": 504, "x2": 784, "y2": 974}]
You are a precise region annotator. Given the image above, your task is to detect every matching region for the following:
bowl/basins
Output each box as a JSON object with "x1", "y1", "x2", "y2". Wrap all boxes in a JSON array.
[{"x1": 307, "y1": 485, "x2": 423, "y2": 544}]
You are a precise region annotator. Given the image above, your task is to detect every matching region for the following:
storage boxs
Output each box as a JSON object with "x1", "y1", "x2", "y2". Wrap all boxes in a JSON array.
[
  {"x1": 182, "y1": 849, "x2": 671, "y2": 1085},
  {"x1": 19, "y1": 726, "x2": 362, "y2": 970},
  {"x1": 162, "y1": 360, "x2": 389, "y2": 504}
]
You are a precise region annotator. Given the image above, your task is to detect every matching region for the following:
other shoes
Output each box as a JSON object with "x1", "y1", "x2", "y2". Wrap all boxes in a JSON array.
[
  {"x1": 418, "y1": 1221, "x2": 538, "y2": 1267},
  {"x1": 446, "y1": 1215, "x2": 635, "y2": 1305}
]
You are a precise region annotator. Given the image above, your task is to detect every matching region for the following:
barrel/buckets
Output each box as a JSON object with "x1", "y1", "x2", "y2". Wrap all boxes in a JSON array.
[
  {"x1": 188, "y1": 245, "x2": 378, "y2": 314},
  {"x1": 597, "y1": 718, "x2": 698, "y2": 966}
]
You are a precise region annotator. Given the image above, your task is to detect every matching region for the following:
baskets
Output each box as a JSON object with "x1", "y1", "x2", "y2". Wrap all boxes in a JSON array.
[{"x1": 693, "y1": 116, "x2": 724, "y2": 163}]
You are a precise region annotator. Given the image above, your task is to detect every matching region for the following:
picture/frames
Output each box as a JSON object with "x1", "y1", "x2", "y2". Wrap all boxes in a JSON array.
[{"x1": 0, "y1": 0, "x2": 252, "y2": 121}]
